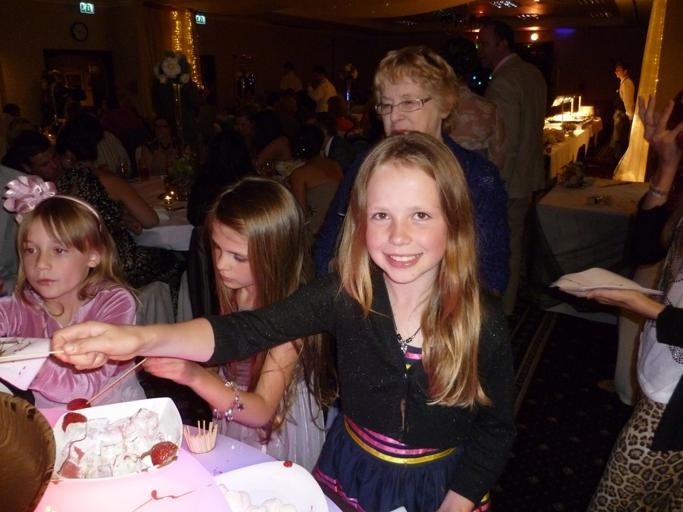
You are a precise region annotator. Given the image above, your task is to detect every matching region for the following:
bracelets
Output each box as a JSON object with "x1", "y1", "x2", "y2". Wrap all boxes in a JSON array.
[
  {"x1": 211, "y1": 380, "x2": 244, "y2": 422},
  {"x1": 648, "y1": 176, "x2": 675, "y2": 199}
]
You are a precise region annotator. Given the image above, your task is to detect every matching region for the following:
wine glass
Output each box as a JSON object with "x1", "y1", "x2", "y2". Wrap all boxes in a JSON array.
[
  {"x1": 119, "y1": 164, "x2": 128, "y2": 176},
  {"x1": 256, "y1": 160, "x2": 273, "y2": 176}
]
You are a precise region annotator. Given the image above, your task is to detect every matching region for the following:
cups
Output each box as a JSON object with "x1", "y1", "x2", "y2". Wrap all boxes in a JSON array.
[{"x1": 140, "y1": 163, "x2": 149, "y2": 181}]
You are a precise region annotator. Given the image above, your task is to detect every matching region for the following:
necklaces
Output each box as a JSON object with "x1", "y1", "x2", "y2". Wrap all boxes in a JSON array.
[{"x1": 395, "y1": 325, "x2": 422, "y2": 354}]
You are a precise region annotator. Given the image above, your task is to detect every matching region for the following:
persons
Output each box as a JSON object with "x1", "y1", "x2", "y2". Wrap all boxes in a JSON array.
[
  {"x1": 441, "y1": 20, "x2": 549, "y2": 317},
  {"x1": 309, "y1": 45, "x2": 509, "y2": 301},
  {"x1": 558, "y1": 87, "x2": 683, "y2": 512},
  {"x1": 609, "y1": 63, "x2": 635, "y2": 144},
  {"x1": 0, "y1": 173, "x2": 148, "y2": 409},
  {"x1": 0, "y1": 57, "x2": 373, "y2": 324},
  {"x1": 141, "y1": 175, "x2": 326, "y2": 473},
  {"x1": 49, "y1": 128, "x2": 515, "y2": 512}
]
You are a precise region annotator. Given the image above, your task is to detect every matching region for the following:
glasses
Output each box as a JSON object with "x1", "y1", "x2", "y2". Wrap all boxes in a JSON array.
[{"x1": 374, "y1": 96, "x2": 433, "y2": 117}]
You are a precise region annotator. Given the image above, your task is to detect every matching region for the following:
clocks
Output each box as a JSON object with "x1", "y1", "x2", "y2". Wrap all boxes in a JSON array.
[{"x1": 72, "y1": 21, "x2": 90, "y2": 41}]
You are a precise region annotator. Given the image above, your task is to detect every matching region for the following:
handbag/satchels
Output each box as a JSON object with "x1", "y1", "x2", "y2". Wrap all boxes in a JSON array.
[{"x1": 614, "y1": 262, "x2": 660, "y2": 408}]
